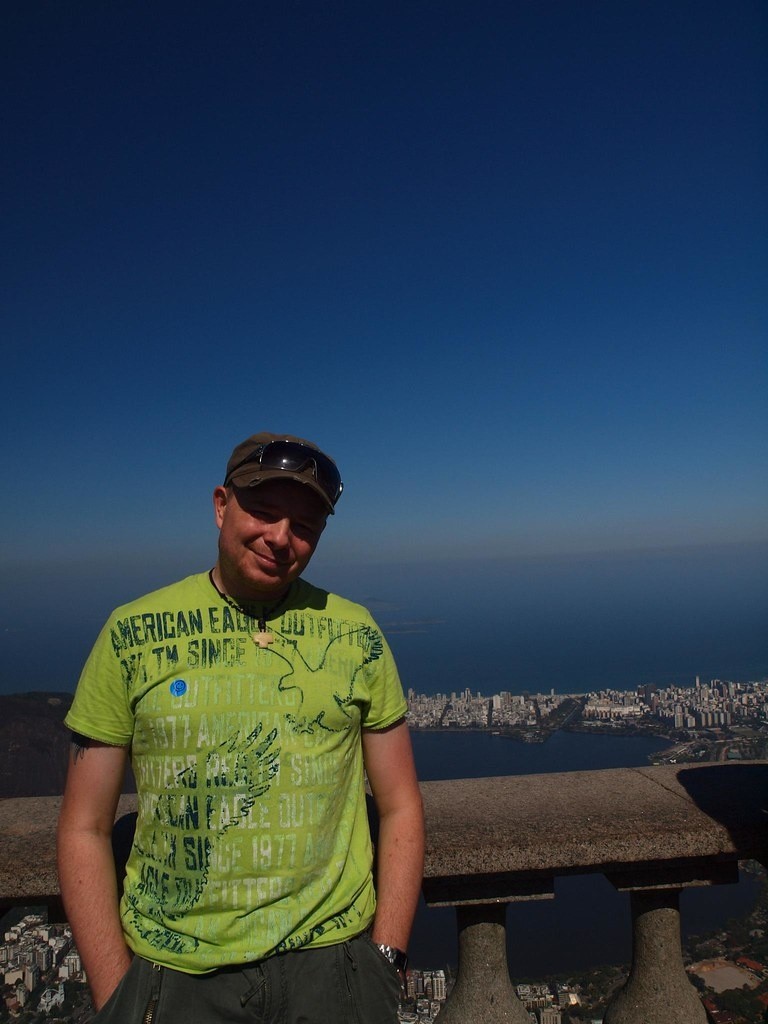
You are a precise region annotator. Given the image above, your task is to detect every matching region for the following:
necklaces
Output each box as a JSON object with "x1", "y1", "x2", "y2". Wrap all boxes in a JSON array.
[{"x1": 208, "y1": 567, "x2": 292, "y2": 649}]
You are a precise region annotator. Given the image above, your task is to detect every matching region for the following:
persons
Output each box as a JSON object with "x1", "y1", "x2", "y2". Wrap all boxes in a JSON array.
[{"x1": 55, "y1": 432, "x2": 423, "y2": 1024}]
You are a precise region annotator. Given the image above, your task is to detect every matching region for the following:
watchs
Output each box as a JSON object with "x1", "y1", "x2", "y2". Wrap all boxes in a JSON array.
[{"x1": 375, "y1": 943, "x2": 408, "y2": 973}]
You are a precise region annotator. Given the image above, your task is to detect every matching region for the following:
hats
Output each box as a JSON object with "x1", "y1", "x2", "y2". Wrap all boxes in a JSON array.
[{"x1": 226, "y1": 431, "x2": 344, "y2": 515}]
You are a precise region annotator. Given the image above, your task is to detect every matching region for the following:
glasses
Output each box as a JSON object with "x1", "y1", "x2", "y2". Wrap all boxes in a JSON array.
[{"x1": 225, "y1": 440, "x2": 345, "y2": 508}]
